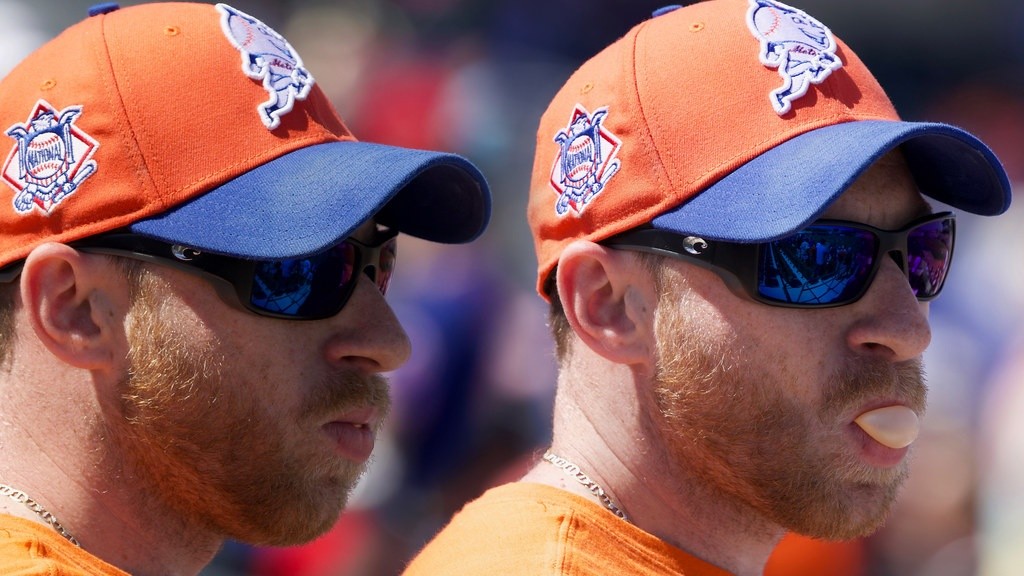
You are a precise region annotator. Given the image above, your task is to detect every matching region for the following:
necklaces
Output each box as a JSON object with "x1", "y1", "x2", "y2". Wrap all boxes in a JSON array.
[
  {"x1": 0, "y1": 484, "x2": 82, "y2": 545},
  {"x1": 531, "y1": 443, "x2": 631, "y2": 523}
]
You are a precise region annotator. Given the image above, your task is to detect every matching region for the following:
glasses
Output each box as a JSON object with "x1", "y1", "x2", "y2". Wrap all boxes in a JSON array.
[
  {"x1": 595, "y1": 211, "x2": 957, "y2": 310},
  {"x1": 1, "y1": 229, "x2": 402, "y2": 321}
]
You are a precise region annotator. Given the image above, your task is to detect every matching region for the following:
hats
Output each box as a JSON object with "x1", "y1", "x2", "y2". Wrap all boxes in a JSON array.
[
  {"x1": 0, "y1": 3, "x2": 493, "y2": 263},
  {"x1": 526, "y1": 0, "x2": 1014, "y2": 304}
]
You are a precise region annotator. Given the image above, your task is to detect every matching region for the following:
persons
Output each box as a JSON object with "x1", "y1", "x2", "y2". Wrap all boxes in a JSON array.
[
  {"x1": 0, "y1": 0, "x2": 492, "y2": 576},
  {"x1": 402, "y1": 0, "x2": 1015, "y2": 575}
]
